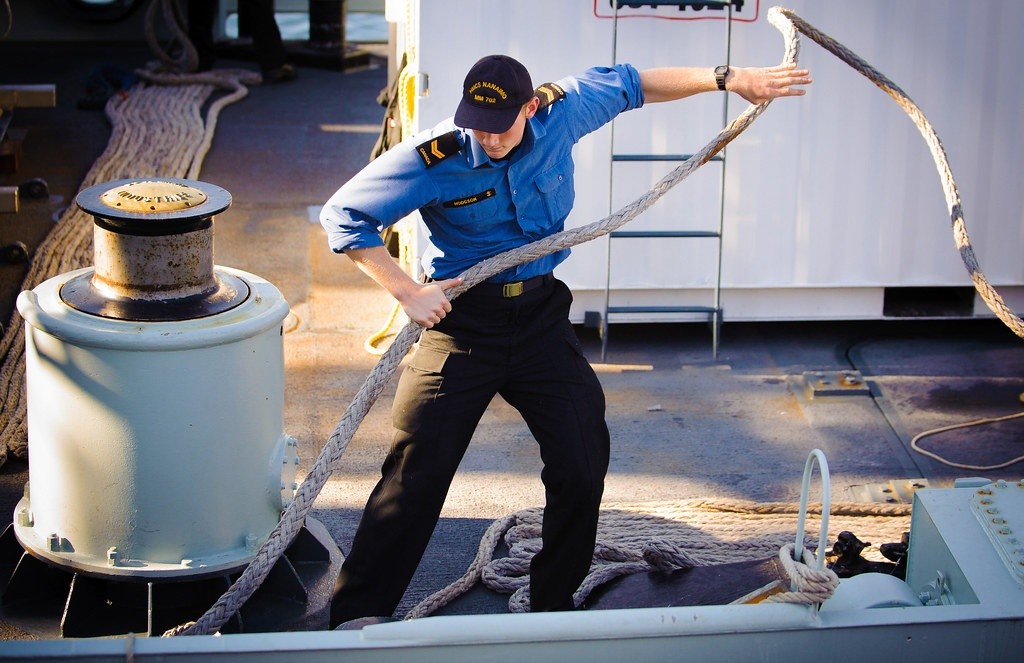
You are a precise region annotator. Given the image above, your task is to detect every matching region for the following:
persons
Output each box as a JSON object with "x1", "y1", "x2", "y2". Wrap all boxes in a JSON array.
[{"x1": 319, "y1": 52, "x2": 813, "y2": 631}]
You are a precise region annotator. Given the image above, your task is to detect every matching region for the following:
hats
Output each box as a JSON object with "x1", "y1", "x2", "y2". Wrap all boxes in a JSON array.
[{"x1": 454, "y1": 55, "x2": 533, "y2": 134}]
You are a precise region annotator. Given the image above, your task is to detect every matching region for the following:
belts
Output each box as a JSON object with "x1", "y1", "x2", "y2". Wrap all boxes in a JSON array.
[{"x1": 473, "y1": 272, "x2": 554, "y2": 298}]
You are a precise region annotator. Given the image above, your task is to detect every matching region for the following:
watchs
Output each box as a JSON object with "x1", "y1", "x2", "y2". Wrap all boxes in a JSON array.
[{"x1": 714, "y1": 66, "x2": 730, "y2": 92}]
